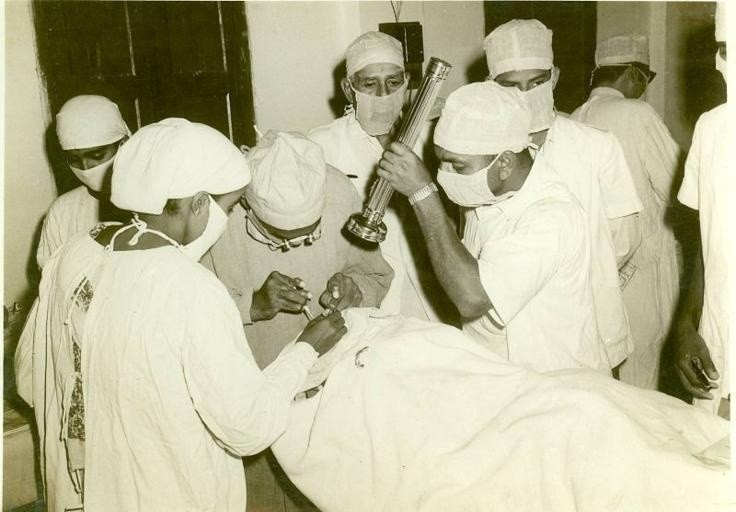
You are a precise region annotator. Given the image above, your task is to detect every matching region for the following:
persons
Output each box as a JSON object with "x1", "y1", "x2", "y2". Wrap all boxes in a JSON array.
[
  {"x1": 476, "y1": 15, "x2": 644, "y2": 384},
  {"x1": 298, "y1": 29, "x2": 463, "y2": 336},
  {"x1": 558, "y1": 31, "x2": 686, "y2": 392},
  {"x1": 33, "y1": 93, "x2": 134, "y2": 272},
  {"x1": 664, "y1": 3, "x2": 731, "y2": 422},
  {"x1": 11, "y1": 116, "x2": 351, "y2": 512},
  {"x1": 373, "y1": 81, "x2": 617, "y2": 388},
  {"x1": 187, "y1": 127, "x2": 398, "y2": 512}
]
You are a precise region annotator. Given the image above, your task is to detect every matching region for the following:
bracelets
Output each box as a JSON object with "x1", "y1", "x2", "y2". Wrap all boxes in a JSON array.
[{"x1": 407, "y1": 182, "x2": 440, "y2": 207}]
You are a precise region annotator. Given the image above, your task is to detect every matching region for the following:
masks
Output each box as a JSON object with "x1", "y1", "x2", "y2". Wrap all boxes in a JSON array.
[
  {"x1": 436, "y1": 150, "x2": 519, "y2": 208},
  {"x1": 67, "y1": 140, "x2": 125, "y2": 193},
  {"x1": 180, "y1": 194, "x2": 230, "y2": 263},
  {"x1": 489, "y1": 69, "x2": 555, "y2": 136},
  {"x1": 347, "y1": 73, "x2": 410, "y2": 137}
]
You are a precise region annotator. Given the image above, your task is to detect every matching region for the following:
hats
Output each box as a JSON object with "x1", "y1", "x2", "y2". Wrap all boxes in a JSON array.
[
  {"x1": 110, "y1": 117, "x2": 251, "y2": 215},
  {"x1": 345, "y1": 31, "x2": 406, "y2": 78},
  {"x1": 243, "y1": 129, "x2": 328, "y2": 231},
  {"x1": 595, "y1": 32, "x2": 651, "y2": 67},
  {"x1": 54, "y1": 92, "x2": 131, "y2": 154},
  {"x1": 482, "y1": 18, "x2": 555, "y2": 79}
]
